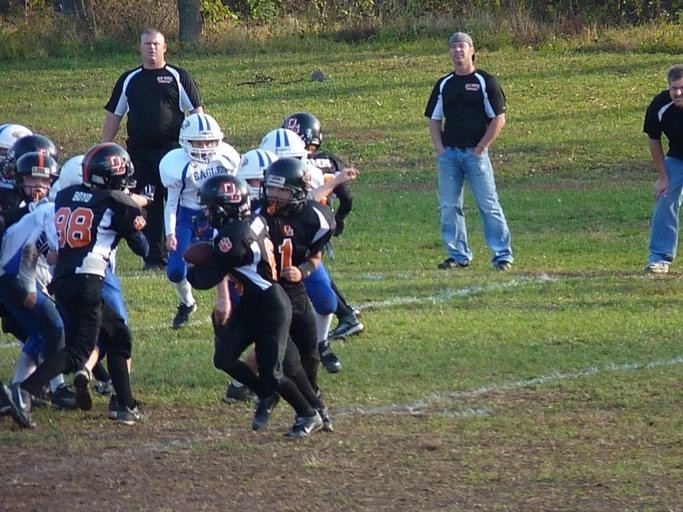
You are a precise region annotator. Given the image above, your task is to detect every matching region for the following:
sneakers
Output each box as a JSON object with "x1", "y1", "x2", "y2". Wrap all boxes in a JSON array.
[
  {"x1": 647, "y1": 263, "x2": 669, "y2": 274},
  {"x1": 143, "y1": 262, "x2": 161, "y2": 271},
  {"x1": 174, "y1": 302, "x2": 198, "y2": 328},
  {"x1": 224, "y1": 340, "x2": 341, "y2": 439},
  {"x1": 439, "y1": 258, "x2": 469, "y2": 270},
  {"x1": 498, "y1": 261, "x2": 512, "y2": 271},
  {"x1": 328, "y1": 304, "x2": 364, "y2": 338},
  {"x1": 0, "y1": 367, "x2": 147, "y2": 429}
]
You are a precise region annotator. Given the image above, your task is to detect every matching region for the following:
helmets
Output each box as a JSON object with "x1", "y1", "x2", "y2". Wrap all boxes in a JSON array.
[
  {"x1": 0, "y1": 124, "x2": 137, "y2": 202},
  {"x1": 179, "y1": 114, "x2": 323, "y2": 228}
]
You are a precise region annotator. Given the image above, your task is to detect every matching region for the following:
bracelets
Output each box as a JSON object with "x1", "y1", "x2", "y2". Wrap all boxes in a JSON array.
[{"x1": 477, "y1": 144, "x2": 488, "y2": 154}]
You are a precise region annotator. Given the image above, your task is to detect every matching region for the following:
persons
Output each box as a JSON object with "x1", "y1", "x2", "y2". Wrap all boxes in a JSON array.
[
  {"x1": 423, "y1": 32, "x2": 514, "y2": 270},
  {"x1": 1, "y1": 113, "x2": 364, "y2": 439},
  {"x1": 100, "y1": 27, "x2": 204, "y2": 272},
  {"x1": 642, "y1": 64, "x2": 682, "y2": 274}
]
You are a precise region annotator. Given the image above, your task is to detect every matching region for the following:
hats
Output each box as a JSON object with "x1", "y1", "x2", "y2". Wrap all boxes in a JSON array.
[{"x1": 449, "y1": 32, "x2": 474, "y2": 61}]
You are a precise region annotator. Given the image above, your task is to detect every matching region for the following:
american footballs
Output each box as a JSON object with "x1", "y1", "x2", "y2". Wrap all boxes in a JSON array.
[{"x1": 183, "y1": 241, "x2": 213, "y2": 266}]
